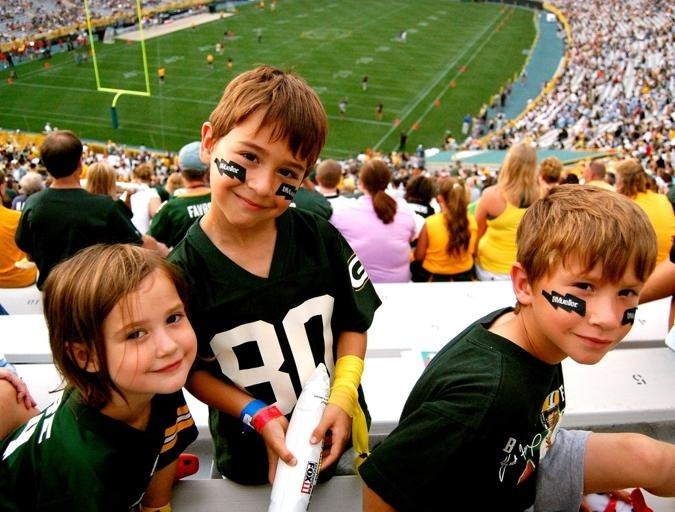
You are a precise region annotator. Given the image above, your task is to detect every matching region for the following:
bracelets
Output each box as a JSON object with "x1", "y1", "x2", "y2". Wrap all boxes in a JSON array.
[{"x1": 240, "y1": 400, "x2": 284, "y2": 431}]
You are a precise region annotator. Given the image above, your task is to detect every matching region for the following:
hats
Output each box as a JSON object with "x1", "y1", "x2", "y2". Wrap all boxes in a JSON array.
[{"x1": 177, "y1": 141, "x2": 209, "y2": 178}]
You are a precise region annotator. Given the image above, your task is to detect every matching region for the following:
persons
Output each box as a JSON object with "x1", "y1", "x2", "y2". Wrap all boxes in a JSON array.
[
  {"x1": 356, "y1": 184, "x2": 675, "y2": 512},
  {"x1": 1, "y1": 1, "x2": 674, "y2": 285},
  {"x1": 638, "y1": 249, "x2": 675, "y2": 353},
  {"x1": 163, "y1": 63, "x2": 381, "y2": 486},
  {"x1": 0, "y1": 244, "x2": 190, "y2": 511},
  {"x1": 0, "y1": 298, "x2": 41, "y2": 442}
]
